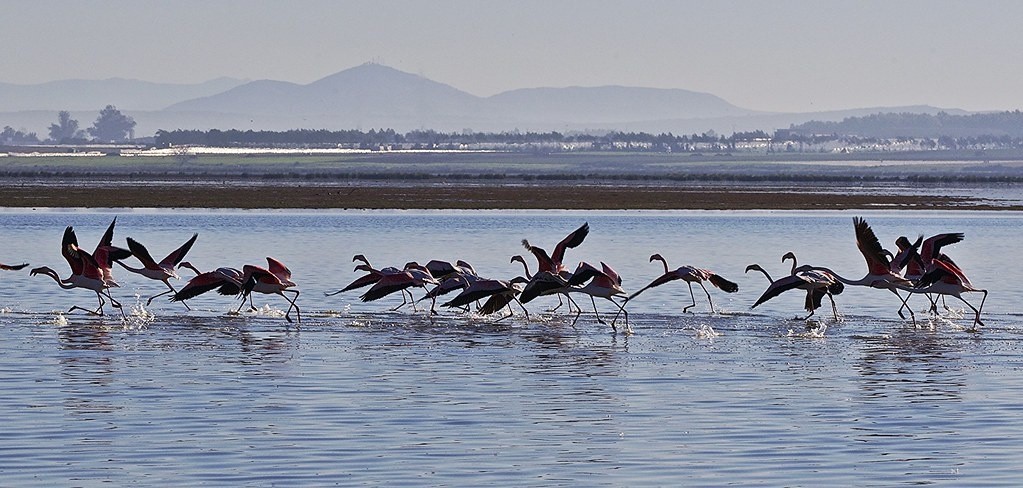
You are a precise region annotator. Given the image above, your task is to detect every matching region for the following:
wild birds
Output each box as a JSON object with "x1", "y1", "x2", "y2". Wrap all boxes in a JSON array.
[{"x1": 1, "y1": 215, "x2": 990, "y2": 335}]
